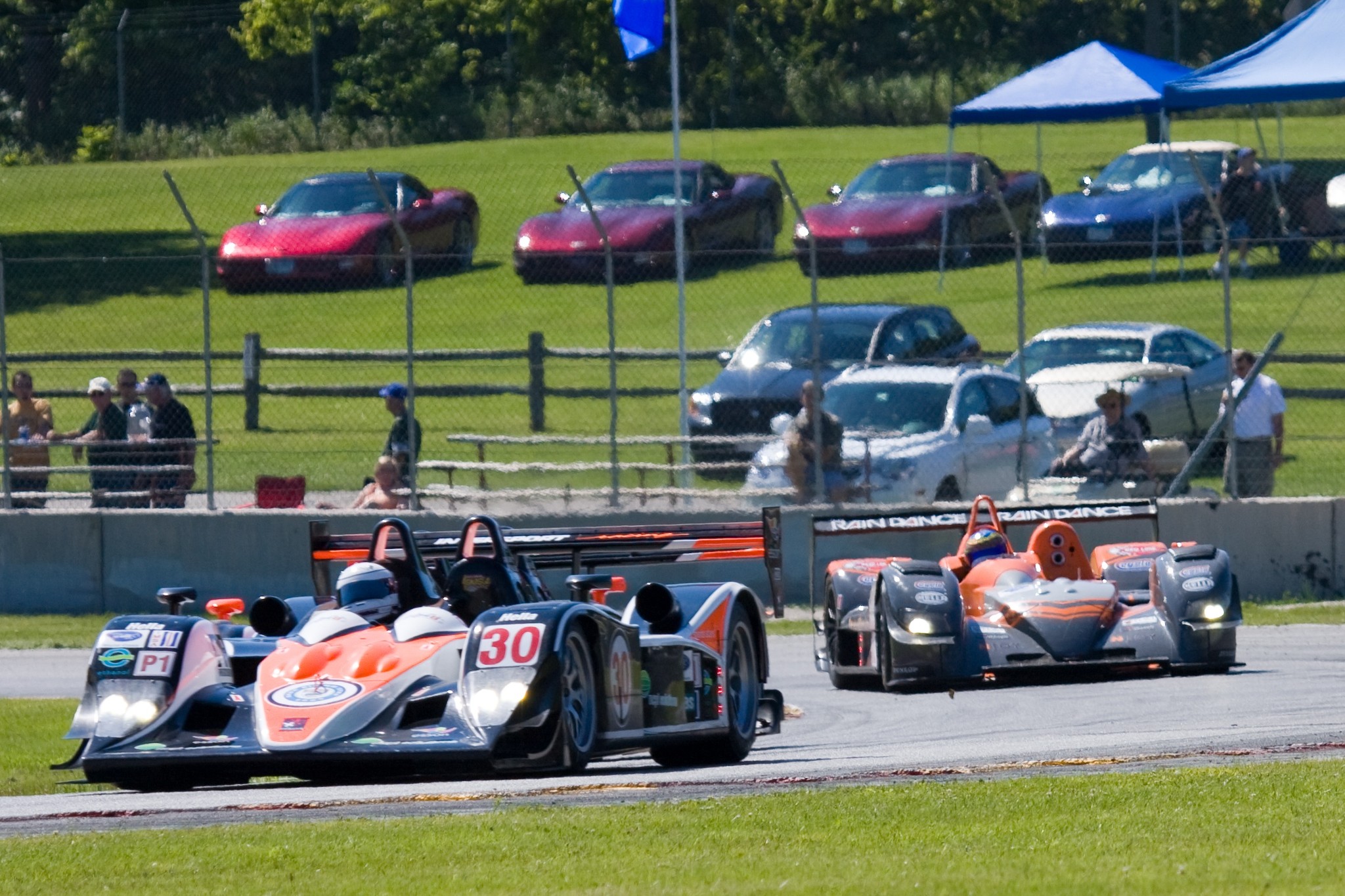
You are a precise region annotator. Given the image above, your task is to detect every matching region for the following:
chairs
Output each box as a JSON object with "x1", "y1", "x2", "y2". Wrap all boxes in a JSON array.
[
  {"x1": 1027, "y1": 521, "x2": 1096, "y2": 580},
  {"x1": 446, "y1": 516, "x2": 530, "y2": 628},
  {"x1": 956, "y1": 494, "x2": 1014, "y2": 560},
  {"x1": 364, "y1": 517, "x2": 451, "y2": 613}
]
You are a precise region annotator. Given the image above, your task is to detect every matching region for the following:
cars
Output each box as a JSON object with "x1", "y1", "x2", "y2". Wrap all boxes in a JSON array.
[
  {"x1": 688, "y1": 302, "x2": 979, "y2": 477},
  {"x1": 742, "y1": 360, "x2": 1059, "y2": 500},
  {"x1": 1250, "y1": 157, "x2": 1345, "y2": 256},
  {"x1": 1004, "y1": 321, "x2": 1249, "y2": 455}
]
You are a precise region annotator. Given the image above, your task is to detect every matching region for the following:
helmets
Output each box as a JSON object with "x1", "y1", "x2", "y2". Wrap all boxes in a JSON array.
[
  {"x1": 965, "y1": 529, "x2": 1008, "y2": 569},
  {"x1": 334, "y1": 561, "x2": 400, "y2": 623}
]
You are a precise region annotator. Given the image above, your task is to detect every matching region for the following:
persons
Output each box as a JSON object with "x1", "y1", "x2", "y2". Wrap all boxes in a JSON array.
[
  {"x1": 1040, "y1": 389, "x2": 1156, "y2": 486},
  {"x1": 336, "y1": 562, "x2": 399, "y2": 624},
  {"x1": 1218, "y1": 349, "x2": 1287, "y2": 499},
  {"x1": 784, "y1": 380, "x2": 850, "y2": 505},
  {"x1": 314, "y1": 384, "x2": 421, "y2": 511},
  {"x1": 0, "y1": 370, "x2": 53, "y2": 509},
  {"x1": 1211, "y1": 147, "x2": 1262, "y2": 278},
  {"x1": 46, "y1": 370, "x2": 197, "y2": 509}
]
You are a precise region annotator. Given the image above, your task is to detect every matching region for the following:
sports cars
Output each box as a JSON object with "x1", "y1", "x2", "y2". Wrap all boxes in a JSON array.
[
  {"x1": 794, "y1": 152, "x2": 1056, "y2": 276},
  {"x1": 1031, "y1": 139, "x2": 1266, "y2": 264},
  {"x1": 512, "y1": 159, "x2": 783, "y2": 282},
  {"x1": 214, "y1": 173, "x2": 481, "y2": 290}
]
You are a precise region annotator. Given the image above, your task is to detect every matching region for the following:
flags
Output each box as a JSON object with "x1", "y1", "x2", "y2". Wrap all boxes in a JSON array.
[{"x1": 612, "y1": 0, "x2": 665, "y2": 61}]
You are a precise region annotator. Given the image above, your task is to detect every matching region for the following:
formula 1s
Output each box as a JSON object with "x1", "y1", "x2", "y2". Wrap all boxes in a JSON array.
[
  {"x1": 805, "y1": 493, "x2": 1248, "y2": 693},
  {"x1": 48, "y1": 513, "x2": 796, "y2": 786}
]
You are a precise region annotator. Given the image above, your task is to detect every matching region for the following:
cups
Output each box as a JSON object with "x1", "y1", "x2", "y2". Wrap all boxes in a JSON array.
[{"x1": 18, "y1": 427, "x2": 30, "y2": 441}]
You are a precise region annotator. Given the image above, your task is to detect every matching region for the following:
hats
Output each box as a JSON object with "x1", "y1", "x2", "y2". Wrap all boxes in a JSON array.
[
  {"x1": 87, "y1": 377, "x2": 111, "y2": 395},
  {"x1": 379, "y1": 382, "x2": 407, "y2": 400},
  {"x1": 1094, "y1": 387, "x2": 1131, "y2": 408},
  {"x1": 135, "y1": 373, "x2": 168, "y2": 393}
]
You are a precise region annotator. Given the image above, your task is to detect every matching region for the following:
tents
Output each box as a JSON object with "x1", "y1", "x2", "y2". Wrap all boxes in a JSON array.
[
  {"x1": 1150, "y1": 0, "x2": 1345, "y2": 282},
  {"x1": 936, "y1": 39, "x2": 1240, "y2": 288}
]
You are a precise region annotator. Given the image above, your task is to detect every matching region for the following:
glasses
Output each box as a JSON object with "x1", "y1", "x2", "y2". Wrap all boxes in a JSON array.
[{"x1": 90, "y1": 391, "x2": 106, "y2": 398}]
